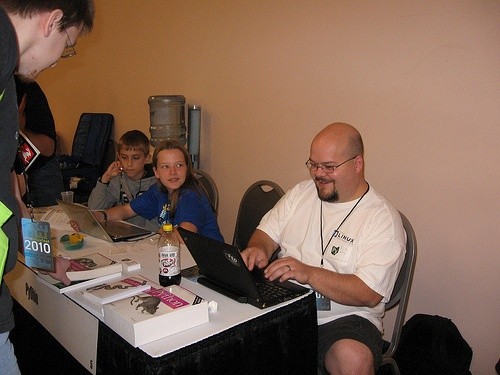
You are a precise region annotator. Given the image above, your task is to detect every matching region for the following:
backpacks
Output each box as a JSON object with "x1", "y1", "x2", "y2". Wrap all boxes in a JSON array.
[{"x1": 395, "y1": 315, "x2": 473, "y2": 375}]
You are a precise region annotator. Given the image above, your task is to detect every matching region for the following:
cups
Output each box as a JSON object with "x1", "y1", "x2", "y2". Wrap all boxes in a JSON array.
[{"x1": 61, "y1": 191, "x2": 74, "y2": 204}]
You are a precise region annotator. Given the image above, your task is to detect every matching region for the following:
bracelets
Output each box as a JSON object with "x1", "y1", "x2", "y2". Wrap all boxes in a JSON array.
[{"x1": 98, "y1": 210, "x2": 109, "y2": 222}]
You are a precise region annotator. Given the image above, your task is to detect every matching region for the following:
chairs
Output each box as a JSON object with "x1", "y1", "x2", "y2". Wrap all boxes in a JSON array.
[
  {"x1": 376, "y1": 209, "x2": 417, "y2": 375},
  {"x1": 60, "y1": 113, "x2": 114, "y2": 203},
  {"x1": 231, "y1": 180, "x2": 285, "y2": 252}
]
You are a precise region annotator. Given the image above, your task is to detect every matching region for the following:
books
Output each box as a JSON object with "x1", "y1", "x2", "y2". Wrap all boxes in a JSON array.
[{"x1": 35, "y1": 253, "x2": 209, "y2": 348}]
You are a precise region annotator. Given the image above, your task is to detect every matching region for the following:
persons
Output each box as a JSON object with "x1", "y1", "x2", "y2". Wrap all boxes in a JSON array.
[
  {"x1": 0, "y1": 0, "x2": 94, "y2": 375},
  {"x1": 13, "y1": 73, "x2": 65, "y2": 207},
  {"x1": 69, "y1": 140, "x2": 225, "y2": 242},
  {"x1": 239, "y1": 122, "x2": 407, "y2": 375},
  {"x1": 88, "y1": 130, "x2": 162, "y2": 232}
]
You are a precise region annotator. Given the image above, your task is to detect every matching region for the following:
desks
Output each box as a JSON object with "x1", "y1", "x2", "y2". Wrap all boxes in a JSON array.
[{"x1": 0, "y1": 204, "x2": 318, "y2": 375}]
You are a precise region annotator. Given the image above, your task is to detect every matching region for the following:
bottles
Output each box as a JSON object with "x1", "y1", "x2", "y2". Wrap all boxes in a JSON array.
[
  {"x1": 148, "y1": 94, "x2": 186, "y2": 150},
  {"x1": 158, "y1": 223, "x2": 181, "y2": 287}
]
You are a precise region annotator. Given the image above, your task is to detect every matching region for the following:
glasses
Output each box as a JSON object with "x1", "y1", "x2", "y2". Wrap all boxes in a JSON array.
[
  {"x1": 61, "y1": 27, "x2": 76, "y2": 58},
  {"x1": 305, "y1": 156, "x2": 356, "y2": 172}
]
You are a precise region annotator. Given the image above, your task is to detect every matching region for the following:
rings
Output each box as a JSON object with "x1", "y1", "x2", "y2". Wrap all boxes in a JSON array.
[{"x1": 286, "y1": 265, "x2": 291, "y2": 271}]
